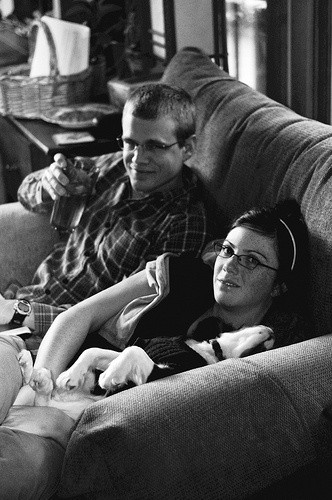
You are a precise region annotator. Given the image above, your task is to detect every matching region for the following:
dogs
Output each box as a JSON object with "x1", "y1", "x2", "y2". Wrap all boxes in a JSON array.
[{"x1": 12, "y1": 323, "x2": 276, "y2": 413}]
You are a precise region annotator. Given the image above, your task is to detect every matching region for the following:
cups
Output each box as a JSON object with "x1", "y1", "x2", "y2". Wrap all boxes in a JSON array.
[{"x1": 50, "y1": 159, "x2": 101, "y2": 233}]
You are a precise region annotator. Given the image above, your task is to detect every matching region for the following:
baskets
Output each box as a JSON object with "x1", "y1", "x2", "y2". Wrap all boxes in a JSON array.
[{"x1": 0, "y1": 15, "x2": 94, "y2": 124}]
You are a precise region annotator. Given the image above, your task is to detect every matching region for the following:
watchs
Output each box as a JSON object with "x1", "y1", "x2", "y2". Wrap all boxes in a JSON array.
[{"x1": 8, "y1": 298, "x2": 32, "y2": 331}]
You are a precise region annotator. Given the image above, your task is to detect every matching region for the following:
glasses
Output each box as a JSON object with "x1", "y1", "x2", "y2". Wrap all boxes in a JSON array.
[
  {"x1": 116, "y1": 134, "x2": 184, "y2": 154},
  {"x1": 213, "y1": 239, "x2": 281, "y2": 275}
]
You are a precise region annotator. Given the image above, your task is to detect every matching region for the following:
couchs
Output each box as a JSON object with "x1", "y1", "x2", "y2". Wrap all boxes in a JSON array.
[{"x1": 0, "y1": 47, "x2": 332, "y2": 500}]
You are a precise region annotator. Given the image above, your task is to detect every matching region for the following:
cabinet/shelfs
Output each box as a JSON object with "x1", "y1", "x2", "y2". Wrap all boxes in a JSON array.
[{"x1": 0, "y1": 110, "x2": 122, "y2": 205}]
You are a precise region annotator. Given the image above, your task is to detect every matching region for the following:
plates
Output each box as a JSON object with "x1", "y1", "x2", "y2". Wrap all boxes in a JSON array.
[{"x1": 41, "y1": 102, "x2": 119, "y2": 129}]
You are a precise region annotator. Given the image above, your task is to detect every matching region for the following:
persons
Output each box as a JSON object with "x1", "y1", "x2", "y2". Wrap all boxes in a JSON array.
[
  {"x1": 0, "y1": 197, "x2": 311, "y2": 500},
  {"x1": 1, "y1": 82, "x2": 213, "y2": 369}
]
